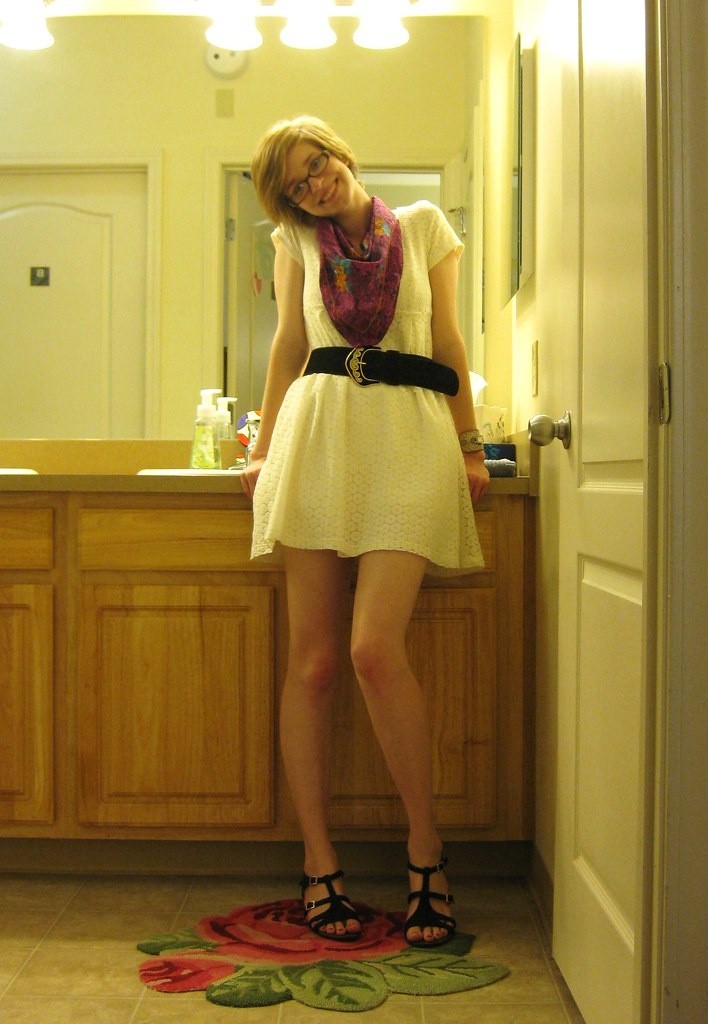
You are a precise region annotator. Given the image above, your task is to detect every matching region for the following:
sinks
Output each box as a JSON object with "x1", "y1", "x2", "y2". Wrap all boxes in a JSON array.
[{"x1": 137, "y1": 467, "x2": 245, "y2": 476}]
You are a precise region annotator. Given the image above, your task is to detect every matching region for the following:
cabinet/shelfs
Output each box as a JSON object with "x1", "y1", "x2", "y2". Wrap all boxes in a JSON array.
[{"x1": 0, "y1": 474, "x2": 535, "y2": 886}]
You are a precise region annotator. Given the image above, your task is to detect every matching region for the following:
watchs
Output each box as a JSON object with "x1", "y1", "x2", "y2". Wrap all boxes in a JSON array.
[{"x1": 458, "y1": 429, "x2": 484, "y2": 453}]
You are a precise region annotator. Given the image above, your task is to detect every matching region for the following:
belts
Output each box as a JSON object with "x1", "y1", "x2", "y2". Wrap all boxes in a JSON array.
[{"x1": 302, "y1": 344, "x2": 459, "y2": 397}]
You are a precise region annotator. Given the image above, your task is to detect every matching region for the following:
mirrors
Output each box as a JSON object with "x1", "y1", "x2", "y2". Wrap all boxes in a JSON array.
[{"x1": 0, "y1": 0, "x2": 493, "y2": 440}]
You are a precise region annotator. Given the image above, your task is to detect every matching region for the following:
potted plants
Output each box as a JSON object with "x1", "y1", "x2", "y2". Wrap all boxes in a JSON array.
[{"x1": 482, "y1": 413, "x2": 518, "y2": 478}]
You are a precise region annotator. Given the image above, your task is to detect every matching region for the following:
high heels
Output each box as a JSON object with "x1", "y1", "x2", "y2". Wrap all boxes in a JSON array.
[
  {"x1": 403, "y1": 856, "x2": 457, "y2": 947},
  {"x1": 299, "y1": 869, "x2": 362, "y2": 941}
]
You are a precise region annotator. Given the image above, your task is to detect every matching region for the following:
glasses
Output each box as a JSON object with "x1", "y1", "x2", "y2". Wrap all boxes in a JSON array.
[{"x1": 287, "y1": 148, "x2": 330, "y2": 208}]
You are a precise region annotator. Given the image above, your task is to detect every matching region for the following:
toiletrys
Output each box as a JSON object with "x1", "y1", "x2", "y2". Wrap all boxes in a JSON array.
[
  {"x1": 191, "y1": 390, "x2": 224, "y2": 468},
  {"x1": 216, "y1": 397, "x2": 238, "y2": 439},
  {"x1": 228, "y1": 451, "x2": 247, "y2": 471}
]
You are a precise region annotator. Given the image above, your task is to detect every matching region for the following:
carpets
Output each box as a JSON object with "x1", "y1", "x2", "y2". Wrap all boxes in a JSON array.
[{"x1": 130, "y1": 896, "x2": 511, "y2": 1011}]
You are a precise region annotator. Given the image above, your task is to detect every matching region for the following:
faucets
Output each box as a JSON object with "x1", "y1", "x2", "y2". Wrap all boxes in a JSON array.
[{"x1": 245, "y1": 418, "x2": 261, "y2": 467}]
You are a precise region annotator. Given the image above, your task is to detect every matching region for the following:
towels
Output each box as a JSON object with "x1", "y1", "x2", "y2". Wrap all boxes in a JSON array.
[{"x1": 484, "y1": 458, "x2": 517, "y2": 478}]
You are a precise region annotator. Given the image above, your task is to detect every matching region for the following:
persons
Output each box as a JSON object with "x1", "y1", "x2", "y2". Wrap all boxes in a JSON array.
[{"x1": 239, "y1": 115, "x2": 490, "y2": 949}]
